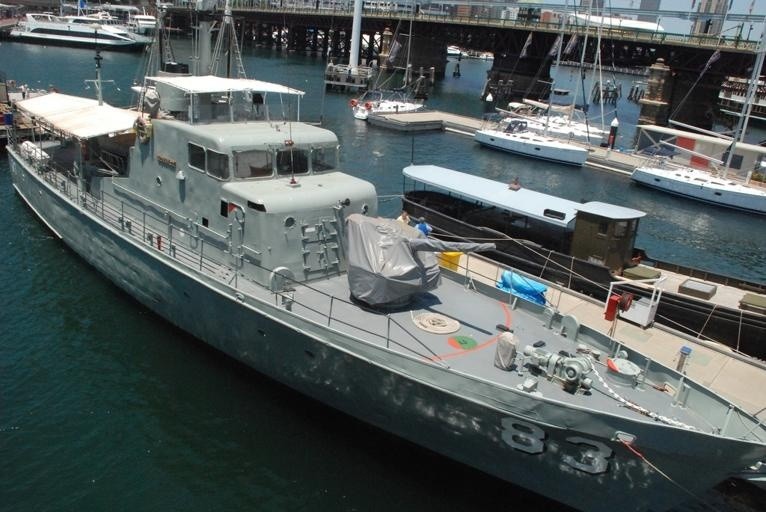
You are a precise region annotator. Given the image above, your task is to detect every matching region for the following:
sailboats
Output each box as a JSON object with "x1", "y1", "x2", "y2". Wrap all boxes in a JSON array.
[
  {"x1": 632, "y1": 31, "x2": 766, "y2": 212},
  {"x1": 352, "y1": 2, "x2": 431, "y2": 120},
  {"x1": 476, "y1": 1, "x2": 610, "y2": 167}
]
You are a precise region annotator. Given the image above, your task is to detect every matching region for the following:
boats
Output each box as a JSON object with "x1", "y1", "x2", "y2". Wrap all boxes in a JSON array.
[
  {"x1": 398, "y1": 165, "x2": 765, "y2": 348},
  {"x1": 10, "y1": 3, "x2": 182, "y2": 48}
]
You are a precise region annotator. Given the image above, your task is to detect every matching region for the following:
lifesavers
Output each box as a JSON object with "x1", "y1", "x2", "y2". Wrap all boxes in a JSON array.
[{"x1": 133, "y1": 116, "x2": 152, "y2": 145}]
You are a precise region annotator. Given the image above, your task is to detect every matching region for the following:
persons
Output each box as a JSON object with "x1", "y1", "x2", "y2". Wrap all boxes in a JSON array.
[
  {"x1": 414, "y1": 216, "x2": 433, "y2": 238},
  {"x1": 16, "y1": 82, "x2": 25, "y2": 101},
  {"x1": 22, "y1": 84, "x2": 30, "y2": 100},
  {"x1": 396, "y1": 211, "x2": 411, "y2": 227}
]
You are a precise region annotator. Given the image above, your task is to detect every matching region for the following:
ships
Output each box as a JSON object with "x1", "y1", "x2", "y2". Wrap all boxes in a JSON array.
[{"x1": 3, "y1": 0, "x2": 766, "y2": 509}]
